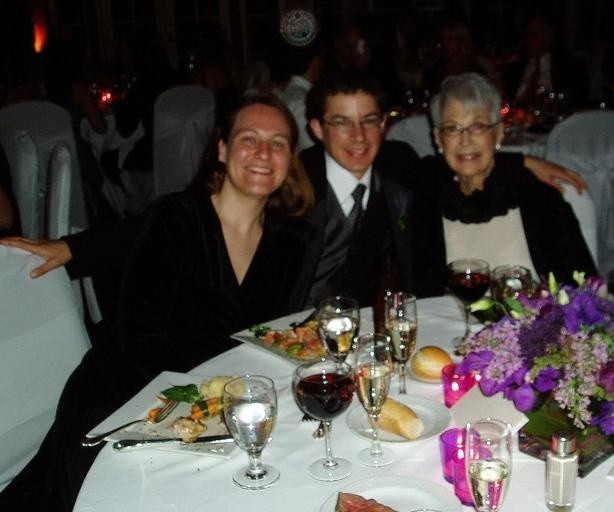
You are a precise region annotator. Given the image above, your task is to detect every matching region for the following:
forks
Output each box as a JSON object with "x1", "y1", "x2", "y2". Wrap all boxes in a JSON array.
[
  {"x1": 80, "y1": 398, "x2": 179, "y2": 446},
  {"x1": 301, "y1": 412, "x2": 317, "y2": 423}
]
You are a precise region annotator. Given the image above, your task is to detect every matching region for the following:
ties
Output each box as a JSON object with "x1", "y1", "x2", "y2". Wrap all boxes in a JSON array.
[{"x1": 347, "y1": 183, "x2": 367, "y2": 230}]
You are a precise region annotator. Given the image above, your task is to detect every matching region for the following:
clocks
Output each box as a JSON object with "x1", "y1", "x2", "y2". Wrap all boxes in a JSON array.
[{"x1": 279, "y1": 9, "x2": 318, "y2": 48}]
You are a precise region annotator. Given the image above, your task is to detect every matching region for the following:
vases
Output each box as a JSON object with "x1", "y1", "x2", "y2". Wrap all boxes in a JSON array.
[{"x1": 514, "y1": 407, "x2": 614, "y2": 477}]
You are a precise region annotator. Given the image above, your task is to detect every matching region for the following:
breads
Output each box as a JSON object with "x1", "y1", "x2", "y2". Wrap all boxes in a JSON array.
[
  {"x1": 412, "y1": 345, "x2": 454, "y2": 380},
  {"x1": 199, "y1": 374, "x2": 241, "y2": 400},
  {"x1": 370, "y1": 396, "x2": 424, "y2": 439}
]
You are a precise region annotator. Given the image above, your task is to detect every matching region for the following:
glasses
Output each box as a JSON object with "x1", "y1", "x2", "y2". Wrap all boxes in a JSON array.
[{"x1": 438, "y1": 118, "x2": 500, "y2": 136}]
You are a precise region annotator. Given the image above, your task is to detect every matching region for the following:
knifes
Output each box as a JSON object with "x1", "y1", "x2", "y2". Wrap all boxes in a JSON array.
[{"x1": 111, "y1": 435, "x2": 233, "y2": 451}]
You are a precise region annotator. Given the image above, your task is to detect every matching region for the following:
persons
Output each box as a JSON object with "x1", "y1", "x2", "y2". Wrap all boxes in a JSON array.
[
  {"x1": 0, "y1": 70, "x2": 590, "y2": 315},
  {"x1": 0, "y1": 1, "x2": 613, "y2": 235},
  {"x1": 0, "y1": 92, "x2": 322, "y2": 509},
  {"x1": 403, "y1": 70, "x2": 606, "y2": 302}
]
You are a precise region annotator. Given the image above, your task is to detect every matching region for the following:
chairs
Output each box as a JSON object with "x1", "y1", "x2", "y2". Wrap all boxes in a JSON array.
[{"x1": 0, "y1": 238, "x2": 89, "y2": 495}]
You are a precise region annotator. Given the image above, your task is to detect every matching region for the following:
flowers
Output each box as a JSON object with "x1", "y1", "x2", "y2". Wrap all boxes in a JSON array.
[{"x1": 428, "y1": 267, "x2": 613, "y2": 455}]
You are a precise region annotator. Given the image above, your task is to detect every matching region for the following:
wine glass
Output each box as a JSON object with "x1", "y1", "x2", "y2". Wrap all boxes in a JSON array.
[
  {"x1": 317, "y1": 297, "x2": 362, "y2": 376},
  {"x1": 445, "y1": 258, "x2": 490, "y2": 346},
  {"x1": 221, "y1": 375, "x2": 280, "y2": 490},
  {"x1": 383, "y1": 291, "x2": 417, "y2": 394},
  {"x1": 293, "y1": 359, "x2": 356, "y2": 482},
  {"x1": 354, "y1": 333, "x2": 394, "y2": 466}
]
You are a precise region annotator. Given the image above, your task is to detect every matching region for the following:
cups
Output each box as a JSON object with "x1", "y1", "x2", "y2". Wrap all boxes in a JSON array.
[
  {"x1": 440, "y1": 415, "x2": 512, "y2": 511},
  {"x1": 489, "y1": 264, "x2": 533, "y2": 303}
]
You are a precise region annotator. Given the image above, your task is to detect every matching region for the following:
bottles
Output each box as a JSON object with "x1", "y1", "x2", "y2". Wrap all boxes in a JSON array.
[{"x1": 543, "y1": 430, "x2": 580, "y2": 512}]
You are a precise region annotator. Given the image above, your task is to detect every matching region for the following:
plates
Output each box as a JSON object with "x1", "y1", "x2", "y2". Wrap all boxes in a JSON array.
[
  {"x1": 84, "y1": 368, "x2": 240, "y2": 459},
  {"x1": 230, "y1": 308, "x2": 323, "y2": 368},
  {"x1": 344, "y1": 393, "x2": 453, "y2": 443},
  {"x1": 320, "y1": 476, "x2": 470, "y2": 511}
]
